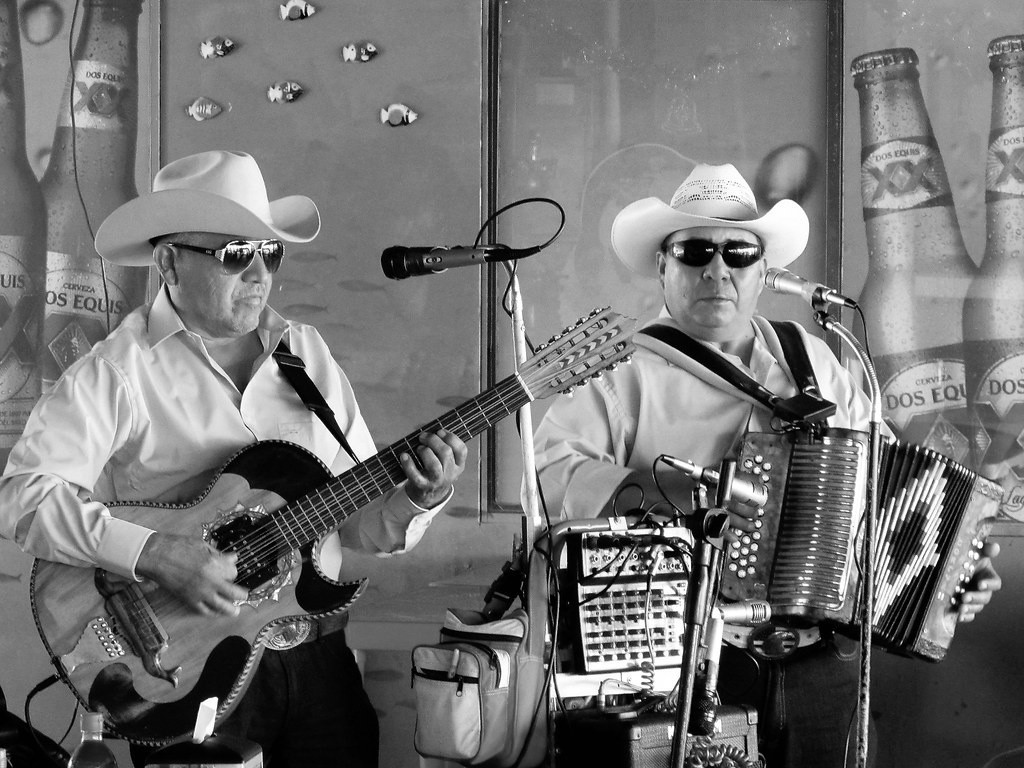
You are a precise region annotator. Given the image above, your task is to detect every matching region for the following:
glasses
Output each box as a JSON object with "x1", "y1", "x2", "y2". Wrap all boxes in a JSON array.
[
  {"x1": 667, "y1": 238, "x2": 764, "y2": 268},
  {"x1": 161, "y1": 240, "x2": 285, "y2": 275}
]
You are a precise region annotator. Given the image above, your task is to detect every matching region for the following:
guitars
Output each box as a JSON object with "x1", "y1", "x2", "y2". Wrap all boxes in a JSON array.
[{"x1": 26, "y1": 303, "x2": 639, "y2": 752}]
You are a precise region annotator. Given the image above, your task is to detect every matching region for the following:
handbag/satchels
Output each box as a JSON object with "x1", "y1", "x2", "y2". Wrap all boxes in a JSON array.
[{"x1": 412, "y1": 548, "x2": 547, "y2": 767}]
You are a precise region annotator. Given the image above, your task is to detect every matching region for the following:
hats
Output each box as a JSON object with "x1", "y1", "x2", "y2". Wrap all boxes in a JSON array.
[
  {"x1": 606, "y1": 164, "x2": 809, "y2": 273},
  {"x1": 94, "y1": 151, "x2": 321, "y2": 266}
]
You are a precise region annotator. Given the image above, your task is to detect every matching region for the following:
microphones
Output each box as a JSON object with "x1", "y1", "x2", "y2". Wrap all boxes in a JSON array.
[
  {"x1": 587, "y1": 534, "x2": 670, "y2": 549},
  {"x1": 711, "y1": 598, "x2": 771, "y2": 623},
  {"x1": 688, "y1": 609, "x2": 724, "y2": 736},
  {"x1": 764, "y1": 267, "x2": 859, "y2": 310},
  {"x1": 483, "y1": 554, "x2": 526, "y2": 623},
  {"x1": 662, "y1": 454, "x2": 768, "y2": 508},
  {"x1": 381, "y1": 244, "x2": 542, "y2": 280}
]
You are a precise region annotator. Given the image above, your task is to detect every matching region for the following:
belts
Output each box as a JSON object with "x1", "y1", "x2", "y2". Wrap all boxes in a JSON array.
[{"x1": 265, "y1": 610, "x2": 349, "y2": 651}]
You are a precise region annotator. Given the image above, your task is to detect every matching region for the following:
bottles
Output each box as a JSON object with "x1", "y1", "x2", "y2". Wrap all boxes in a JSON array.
[
  {"x1": 851, "y1": 47, "x2": 978, "y2": 476},
  {"x1": 38, "y1": 0, "x2": 150, "y2": 398},
  {"x1": 0, "y1": 0, "x2": 48, "y2": 476},
  {"x1": 962, "y1": 34, "x2": 1024, "y2": 524},
  {"x1": 0, "y1": 748, "x2": 7, "y2": 768},
  {"x1": 67, "y1": 712, "x2": 118, "y2": 768}
]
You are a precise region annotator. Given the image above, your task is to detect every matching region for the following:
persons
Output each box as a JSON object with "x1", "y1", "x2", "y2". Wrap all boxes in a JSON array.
[
  {"x1": 517, "y1": 165, "x2": 1003, "y2": 768},
  {"x1": 0, "y1": 148, "x2": 470, "y2": 768}
]
